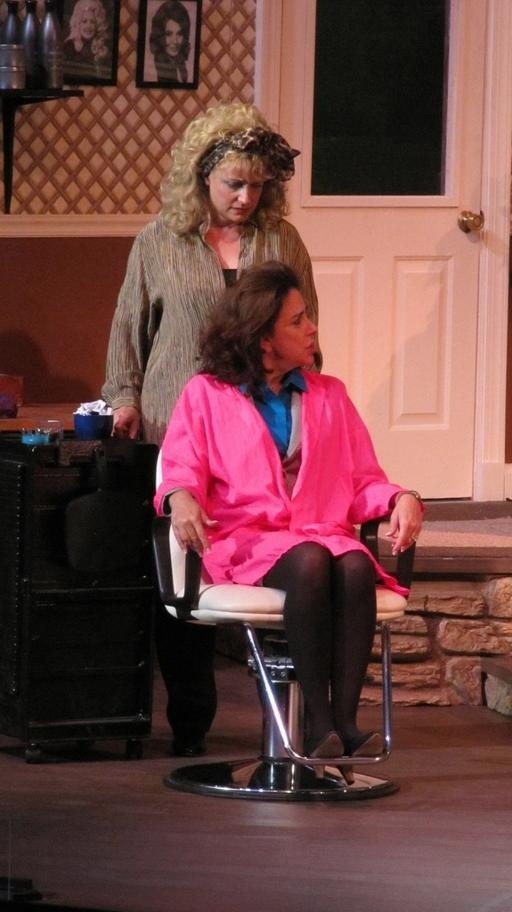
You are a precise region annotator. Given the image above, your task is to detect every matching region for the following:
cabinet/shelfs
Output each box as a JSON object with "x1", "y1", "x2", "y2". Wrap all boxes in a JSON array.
[{"x1": 3, "y1": 431, "x2": 161, "y2": 762}]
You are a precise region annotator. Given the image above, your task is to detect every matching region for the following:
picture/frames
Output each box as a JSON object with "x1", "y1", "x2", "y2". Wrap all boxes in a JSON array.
[{"x1": 43, "y1": 0, "x2": 202, "y2": 89}]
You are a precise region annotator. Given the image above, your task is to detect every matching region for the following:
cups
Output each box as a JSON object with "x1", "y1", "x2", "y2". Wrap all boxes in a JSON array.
[{"x1": 20, "y1": 419, "x2": 63, "y2": 446}]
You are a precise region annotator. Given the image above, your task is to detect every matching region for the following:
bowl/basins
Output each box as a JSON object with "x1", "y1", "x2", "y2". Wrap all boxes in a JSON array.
[{"x1": 74, "y1": 413, "x2": 113, "y2": 440}]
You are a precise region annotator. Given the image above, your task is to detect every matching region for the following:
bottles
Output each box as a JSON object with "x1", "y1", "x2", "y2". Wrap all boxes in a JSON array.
[{"x1": 0, "y1": 2, "x2": 66, "y2": 97}]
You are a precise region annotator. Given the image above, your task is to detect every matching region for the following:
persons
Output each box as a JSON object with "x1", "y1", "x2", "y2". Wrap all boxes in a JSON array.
[
  {"x1": 142, "y1": 0, "x2": 194, "y2": 84},
  {"x1": 99, "y1": 102, "x2": 323, "y2": 758},
  {"x1": 61, "y1": 0, "x2": 112, "y2": 67},
  {"x1": 152, "y1": 260, "x2": 427, "y2": 784}
]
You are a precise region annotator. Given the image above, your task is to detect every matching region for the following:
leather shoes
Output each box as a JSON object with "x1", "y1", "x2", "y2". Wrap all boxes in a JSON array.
[{"x1": 172, "y1": 732, "x2": 208, "y2": 757}]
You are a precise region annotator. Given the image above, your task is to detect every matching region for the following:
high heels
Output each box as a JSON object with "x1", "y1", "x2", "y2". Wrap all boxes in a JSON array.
[
  {"x1": 304, "y1": 730, "x2": 345, "y2": 779},
  {"x1": 336, "y1": 731, "x2": 385, "y2": 786}
]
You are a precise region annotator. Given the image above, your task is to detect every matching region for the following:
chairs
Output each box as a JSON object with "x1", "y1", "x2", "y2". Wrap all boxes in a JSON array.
[{"x1": 146, "y1": 504, "x2": 416, "y2": 804}]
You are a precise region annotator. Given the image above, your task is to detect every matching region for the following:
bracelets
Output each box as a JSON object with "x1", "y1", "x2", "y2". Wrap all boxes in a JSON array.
[{"x1": 396, "y1": 489, "x2": 422, "y2": 503}]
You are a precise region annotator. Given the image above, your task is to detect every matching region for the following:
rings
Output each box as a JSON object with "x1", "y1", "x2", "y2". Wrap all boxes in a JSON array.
[{"x1": 411, "y1": 532, "x2": 419, "y2": 543}]
